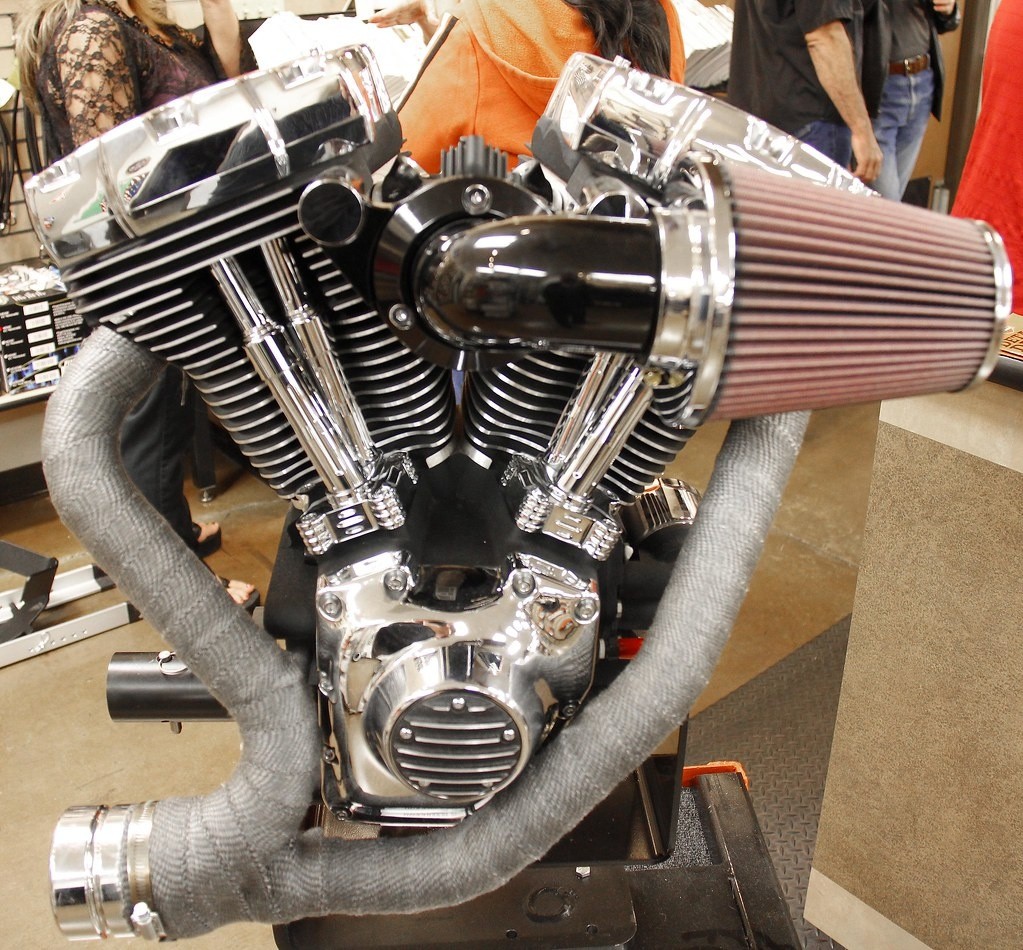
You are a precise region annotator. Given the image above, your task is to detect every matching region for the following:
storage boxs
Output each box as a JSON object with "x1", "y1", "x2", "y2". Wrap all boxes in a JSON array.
[{"x1": 0, "y1": 292, "x2": 89, "y2": 396}]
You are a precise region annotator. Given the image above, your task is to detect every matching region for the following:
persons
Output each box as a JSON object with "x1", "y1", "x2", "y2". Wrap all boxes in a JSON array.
[
  {"x1": 329, "y1": 74, "x2": 356, "y2": 117},
  {"x1": 367, "y1": 0, "x2": 687, "y2": 175},
  {"x1": 373, "y1": 619, "x2": 456, "y2": 658},
  {"x1": 850, "y1": 0, "x2": 961, "y2": 202},
  {"x1": 726, "y1": 0, "x2": 882, "y2": 183},
  {"x1": 17, "y1": 0, "x2": 262, "y2": 617}
]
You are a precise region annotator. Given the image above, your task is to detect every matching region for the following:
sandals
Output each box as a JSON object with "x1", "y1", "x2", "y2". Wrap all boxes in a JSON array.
[
  {"x1": 187, "y1": 522, "x2": 225, "y2": 556},
  {"x1": 217, "y1": 575, "x2": 262, "y2": 616}
]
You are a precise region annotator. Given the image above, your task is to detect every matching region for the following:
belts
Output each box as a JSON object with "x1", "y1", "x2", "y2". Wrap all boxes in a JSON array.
[{"x1": 888, "y1": 54, "x2": 932, "y2": 75}]
